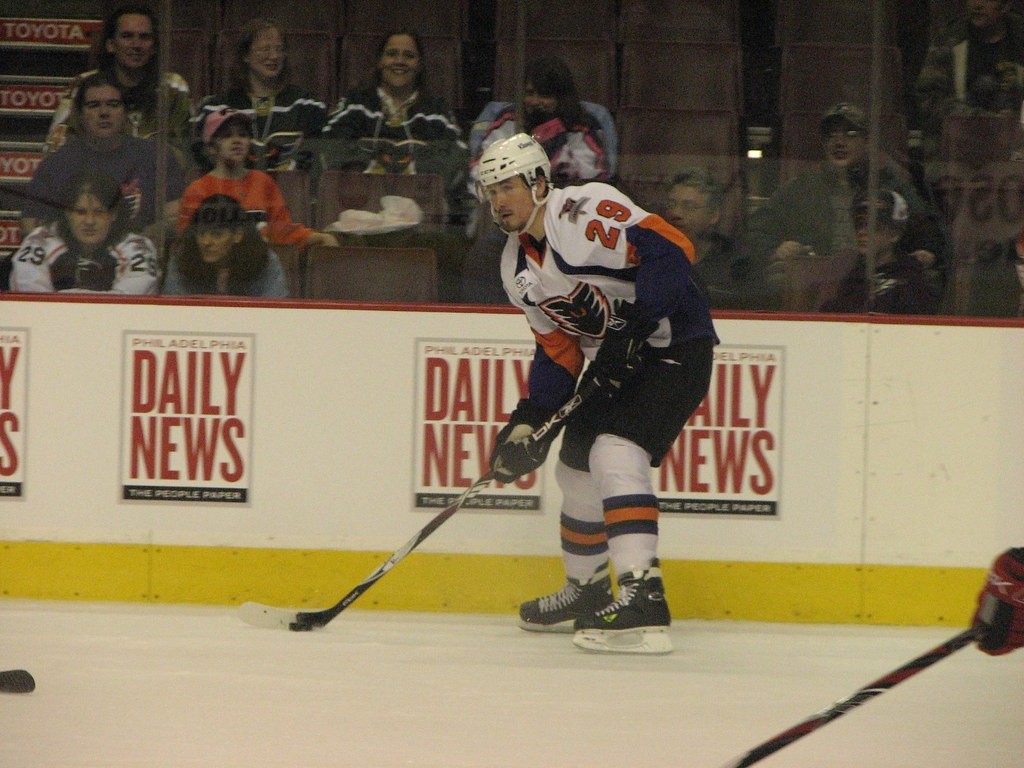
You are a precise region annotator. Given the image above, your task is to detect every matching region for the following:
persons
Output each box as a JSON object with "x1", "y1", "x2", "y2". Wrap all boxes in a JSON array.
[
  {"x1": 916, "y1": 0, "x2": 1024, "y2": 116},
  {"x1": 43, "y1": 0, "x2": 194, "y2": 169},
  {"x1": 473, "y1": 52, "x2": 612, "y2": 179},
  {"x1": 325, "y1": 27, "x2": 469, "y2": 176},
  {"x1": 190, "y1": 18, "x2": 328, "y2": 171},
  {"x1": 814, "y1": 189, "x2": 939, "y2": 317},
  {"x1": 473, "y1": 130, "x2": 719, "y2": 655},
  {"x1": 17, "y1": 74, "x2": 185, "y2": 235},
  {"x1": 173, "y1": 105, "x2": 340, "y2": 248},
  {"x1": 732, "y1": 102, "x2": 925, "y2": 263},
  {"x1": 963, "y1": 221, "x2": 1024, "y2": 320},
  {"x1": 667, "y1": 166, "x2": 792, "y2": 315},
  {"x1": 162, "y1": 192, "x2": 288, "y2": 298},
  {"x1": 7, "y1": 161, "x2": 164, "y2": 297}
]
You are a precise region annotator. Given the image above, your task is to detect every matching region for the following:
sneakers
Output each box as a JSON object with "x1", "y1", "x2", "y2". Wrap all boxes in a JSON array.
[
  {"x1": 517, "y1": 558, "x2": 614, "y2": 633},
  {"x1": 573, "y1": 557, "x2": 674, "y2": 653}
]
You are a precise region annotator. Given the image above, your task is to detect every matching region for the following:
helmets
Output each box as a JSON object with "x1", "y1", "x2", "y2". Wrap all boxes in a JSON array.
[{"x1": 478, "y1": 131, "x2": 551, "y2": 190}]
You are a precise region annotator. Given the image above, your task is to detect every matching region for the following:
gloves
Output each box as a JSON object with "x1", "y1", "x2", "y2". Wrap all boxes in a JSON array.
[
  {"x1": 973, "y1": 547, "x2": 1024, "y2": 657},
  {"x1": 566, "y1": 327, "x2": 655, "y2": 425},
  {"x1": 487, "y1": 395, "x2": 564, "y2": 482}
]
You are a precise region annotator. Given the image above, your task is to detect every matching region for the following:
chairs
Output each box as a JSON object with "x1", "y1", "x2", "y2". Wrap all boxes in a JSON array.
[{"x1": 142, "y1": 0, "x2": 1024, "y2": 319}]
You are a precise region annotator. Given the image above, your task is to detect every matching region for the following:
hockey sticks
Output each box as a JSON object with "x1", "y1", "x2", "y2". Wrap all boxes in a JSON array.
[
  {"x1": 0, "y1": 668, "x2": 36, "y2": 693},
  {"x1": 236, "y1": 375, "x2": 606, "y2": 632},
  {"x1": 727, "y1": 629, "x2": 975, "y2": 768}
]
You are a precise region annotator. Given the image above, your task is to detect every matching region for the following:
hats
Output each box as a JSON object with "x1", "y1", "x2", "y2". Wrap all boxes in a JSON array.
[
  {"x1": 818, "y1": 101, "x2": 868, "y2": 133},
  {"x1": 203, "y1": 108, "x2": 249, "y2": 146},
  {"x1": 850, "y1": 188, "x2": 911, "y2": 232}
]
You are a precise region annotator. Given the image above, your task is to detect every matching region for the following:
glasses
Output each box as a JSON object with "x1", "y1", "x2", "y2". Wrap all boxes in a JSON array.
[{"x1": 825, "y1": 127, "x2": 866, "y2": 142}]
